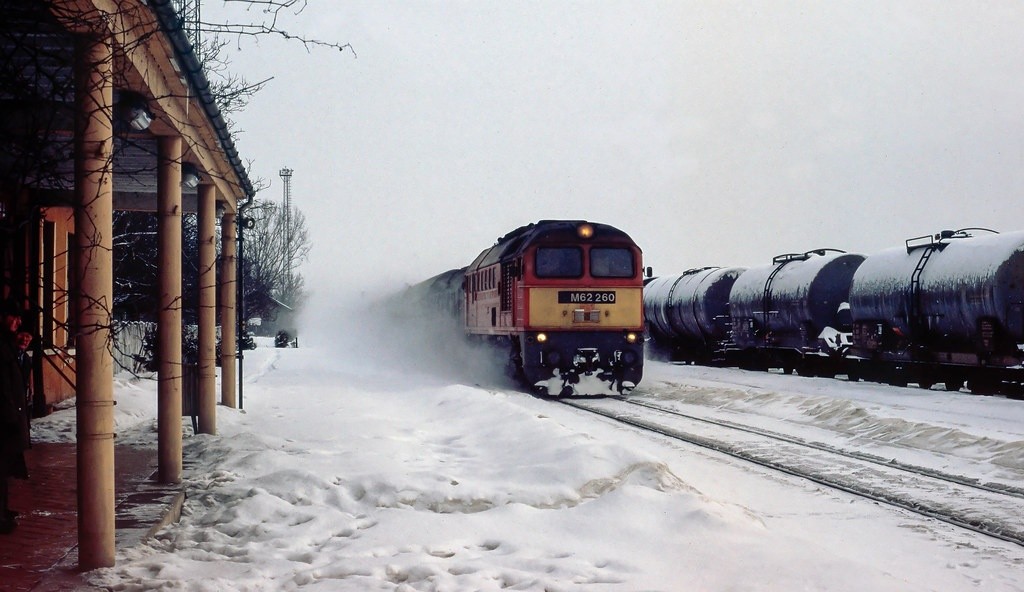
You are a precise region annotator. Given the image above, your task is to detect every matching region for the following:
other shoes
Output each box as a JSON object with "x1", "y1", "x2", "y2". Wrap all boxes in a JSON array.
[{"x1": 0, "y1": 509, "x2": 20, "y2": 531}]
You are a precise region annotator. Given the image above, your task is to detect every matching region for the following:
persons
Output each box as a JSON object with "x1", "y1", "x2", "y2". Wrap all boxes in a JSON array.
[{"x1": 0, "y1": 259, "x2": 38, "y2": 534}]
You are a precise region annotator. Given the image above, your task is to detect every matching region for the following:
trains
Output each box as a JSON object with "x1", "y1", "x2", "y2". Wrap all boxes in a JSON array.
[
  {"x1": 643, "y1": 227, "x2": 1023, "y2": 402},
  {"x1": 375, "y1": 219, "x2": 653, "y2": 401}
]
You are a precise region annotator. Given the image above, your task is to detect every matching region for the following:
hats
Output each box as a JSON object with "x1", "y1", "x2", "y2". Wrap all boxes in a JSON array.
[
  {"x1": 0, "y1": 299, "x2": 29, "y2": 317},
  {"x1": 18, "y1": 319, "x2": 36, "y2": 335}
]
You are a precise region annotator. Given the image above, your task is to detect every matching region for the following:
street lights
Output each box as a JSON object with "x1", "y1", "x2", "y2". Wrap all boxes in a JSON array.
[{"x1": 279, "y1": 165, "x2": 294, "y2": 330}]
